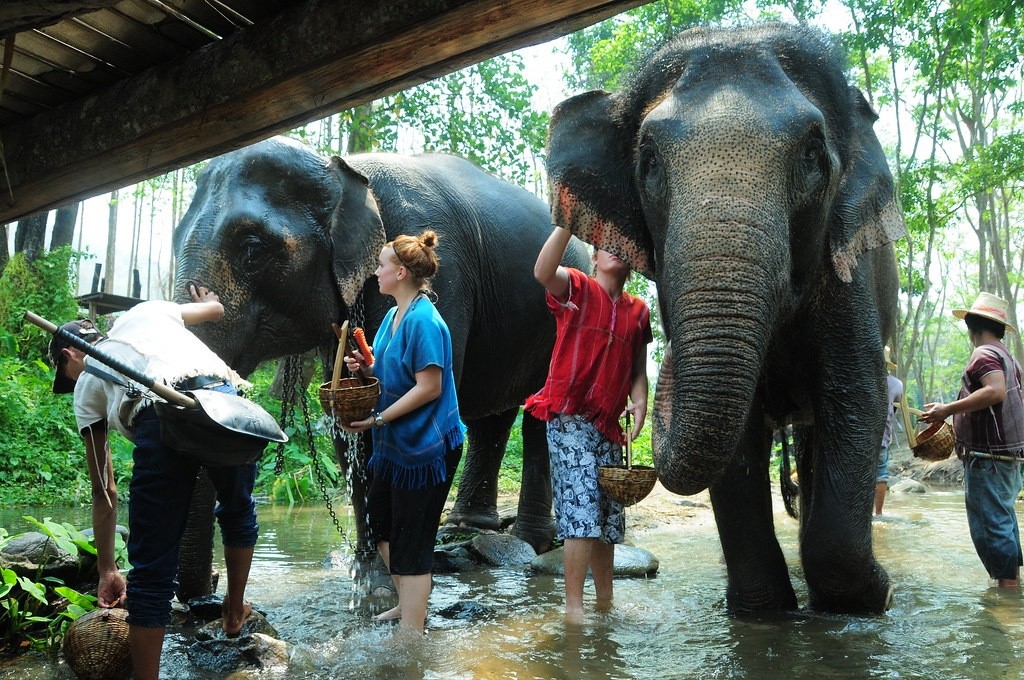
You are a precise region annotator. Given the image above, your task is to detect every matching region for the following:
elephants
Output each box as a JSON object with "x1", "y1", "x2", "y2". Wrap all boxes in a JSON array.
[
  {"x1": 544, "y1": 23, "x2": 899, "y2": 616},
  {"x1": 173, "y1": 133, "x2": 591, "y2": 601}
]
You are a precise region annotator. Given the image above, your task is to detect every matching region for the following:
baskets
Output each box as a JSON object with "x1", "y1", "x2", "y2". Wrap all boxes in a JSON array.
[
  {"x1": 598, "y1": 409, "x2": 657, "y2": 508},
  {"x1": 319, "y1": 320, "x2": 381, "y2": 429},
  {"x1": 63, "y1": 598, "x2": 134, "y2": 680},
  {"x1": 891, "y1": 393, "x2": 955, "y2": 462}
]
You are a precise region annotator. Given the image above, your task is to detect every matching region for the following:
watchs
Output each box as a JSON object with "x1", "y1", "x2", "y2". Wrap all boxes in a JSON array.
[{"x1": 372, "y1": 412, "x2": 386, "y2": 427}]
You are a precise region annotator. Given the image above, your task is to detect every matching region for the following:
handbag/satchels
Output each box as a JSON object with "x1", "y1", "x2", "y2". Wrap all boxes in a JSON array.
[{"x1": 150, "y1": 389, "x2": 289, "y2": 468}]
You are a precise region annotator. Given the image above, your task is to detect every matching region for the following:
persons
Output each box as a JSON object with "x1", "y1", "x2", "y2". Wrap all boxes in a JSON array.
[
  {"x1": 49, "y1": 283, "x2": 268, "y2": 680},
  {"x1": 533, "y1": 226, "x2": 654, "y2": 625},
  {"x1": 920, "y1": 291, "x2": 1023, "y2": 587},
  {"x1": 875, "y1": 346, "x2": 903, "y2": 514},
  {"x1": 335, "y1": 229, "x2": 469, "y2": 635}
]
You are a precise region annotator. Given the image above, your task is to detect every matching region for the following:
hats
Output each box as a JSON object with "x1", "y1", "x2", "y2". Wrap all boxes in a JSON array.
[
  {"x1": 951, "y1": 291, "x2": 1018, "y2": 334},
  {"x1": 883, "y1": 346, "x2": 898, "y2": 369},
  {"x1": 48, "y1": 319, "x2": 103, "y2": 394}
]
